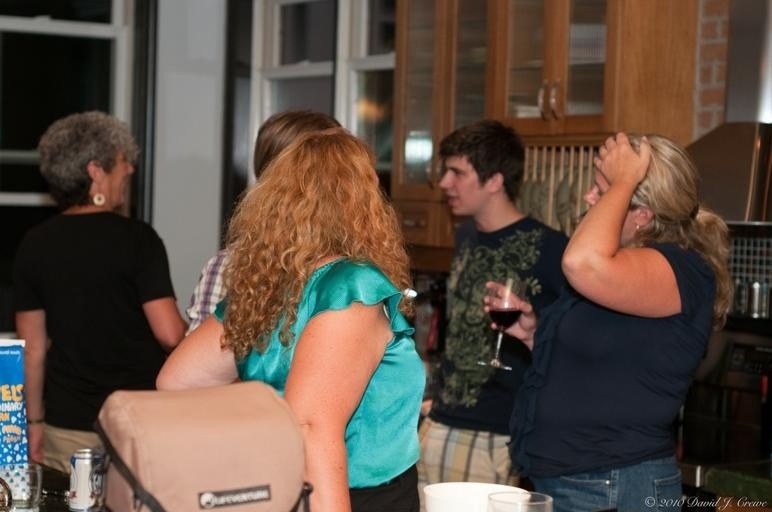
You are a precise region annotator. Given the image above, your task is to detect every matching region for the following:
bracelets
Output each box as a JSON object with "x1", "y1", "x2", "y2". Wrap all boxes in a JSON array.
[{"x1": 27, "y1": 416, "x2": 46, "y2": 425}]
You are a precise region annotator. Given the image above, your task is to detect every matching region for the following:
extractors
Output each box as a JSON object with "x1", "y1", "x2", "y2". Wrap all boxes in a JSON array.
[{"x1": 680, "y1": 2, "x2": 770, "y2": 228}]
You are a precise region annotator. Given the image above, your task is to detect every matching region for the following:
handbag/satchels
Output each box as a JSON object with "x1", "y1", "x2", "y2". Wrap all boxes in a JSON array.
[{"x1": 94, "y1": 378, "x2": 312, "y2": 512}]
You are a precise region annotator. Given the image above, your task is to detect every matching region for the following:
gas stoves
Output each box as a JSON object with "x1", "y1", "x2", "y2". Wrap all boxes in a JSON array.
[{"x1": 726, "y1": 340, "x2": 771, "y2": 373}]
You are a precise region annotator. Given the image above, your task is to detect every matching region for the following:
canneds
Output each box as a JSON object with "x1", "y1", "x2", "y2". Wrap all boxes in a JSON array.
[
  {"x1": 746, "y1": 281, "x2": 766, "y2": 318},
  {"x1": 68, "y1": 447, "x2": 105, "y2": 511}
]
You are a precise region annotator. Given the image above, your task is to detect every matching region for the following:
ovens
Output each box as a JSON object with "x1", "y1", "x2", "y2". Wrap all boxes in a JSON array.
[{"x1": 683, "y1": 376, "x2": 772, "y2": 505}]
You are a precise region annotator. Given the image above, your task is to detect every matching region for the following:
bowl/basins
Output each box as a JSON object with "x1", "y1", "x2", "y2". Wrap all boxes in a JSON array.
[{"x1": 422, "y1": 481, "x2": 531, "y2": 512}]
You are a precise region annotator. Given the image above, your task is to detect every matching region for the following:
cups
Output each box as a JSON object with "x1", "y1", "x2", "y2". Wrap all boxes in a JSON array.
[
  {"x1": 489, "y1": 491, "x2": 554, "y2": 512},
  {"x1": 4, "y1": 461, "x2": 43, "y2": 512},
  {"x1": 747, "y1": 277, "x2": 770, "y2": 319}
]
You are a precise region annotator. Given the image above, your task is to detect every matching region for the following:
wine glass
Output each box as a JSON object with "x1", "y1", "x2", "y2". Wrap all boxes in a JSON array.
[{"x1": 476, "y1": 276, "x2": 528, "y2": 369}]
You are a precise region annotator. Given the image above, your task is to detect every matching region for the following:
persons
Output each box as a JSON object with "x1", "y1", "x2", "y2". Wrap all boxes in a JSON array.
[
  {"x1": 483, "y1": 132, "x2": 734, "y2": 512},
  {"x1": 154, "y1": 128, "x2": 426, "y2": 512},
  {"x1": 184, "y1": 110, "x2": 341, "y2": 338},
  {"x1": 415, "y1": 121, "x2": 569, "y2": 512},
  {"x1": 13, "y1": 110, "x2": 188, "y2": 476}
]
